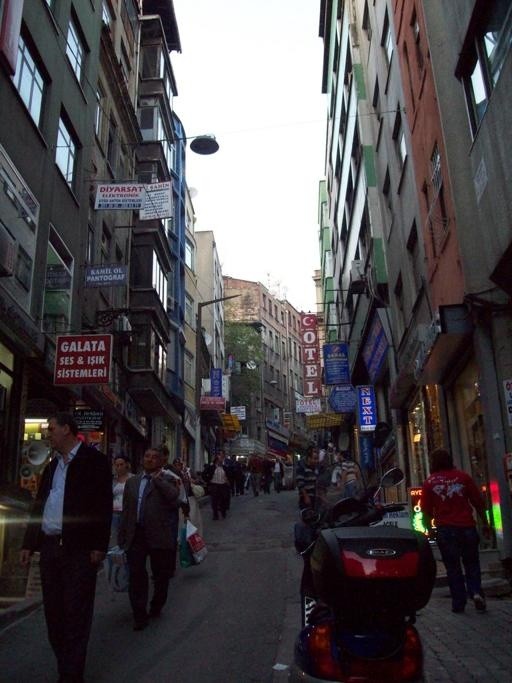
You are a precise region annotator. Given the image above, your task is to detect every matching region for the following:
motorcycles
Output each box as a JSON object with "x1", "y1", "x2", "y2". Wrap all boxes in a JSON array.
[
  {"x1": 258, "y1": 469, "x2": 274, "y2": 495},
  {"x1": 292, "y1": 465, "x2": 437, "y2": 682}
]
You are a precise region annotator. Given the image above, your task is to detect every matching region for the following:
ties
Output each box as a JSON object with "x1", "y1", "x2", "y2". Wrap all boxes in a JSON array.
[{"x1": 139, "y1": 474, "x2": 151, "y2": 530}]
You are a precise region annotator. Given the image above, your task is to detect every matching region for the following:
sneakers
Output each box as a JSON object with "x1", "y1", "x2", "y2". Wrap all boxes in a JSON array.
[
  {"x1": 473, "y1": 591, "x2": 486, "y2": 614},
  {"x1": 450, "y1": 603, "x2": 464, "y2": 613},
  {"x1": 133, "y1": 608, "x2": 160, "y2": 631}
]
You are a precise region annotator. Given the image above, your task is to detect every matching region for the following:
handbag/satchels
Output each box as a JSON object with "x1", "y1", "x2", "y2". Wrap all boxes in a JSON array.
[{"x1": 179, "y1": 520, "x2": 206, "y2": 569}]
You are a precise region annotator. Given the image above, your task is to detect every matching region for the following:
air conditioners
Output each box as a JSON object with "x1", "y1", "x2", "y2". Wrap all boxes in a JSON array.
[{"x1": 349, "y1": 258, "x2": 367, "y2": 293}]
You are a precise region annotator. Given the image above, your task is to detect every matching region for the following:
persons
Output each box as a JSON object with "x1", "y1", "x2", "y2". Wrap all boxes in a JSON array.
[
  {"x1": 18, "y1": 410, "x2": 112, "y2": 682},
  {"x1": 420, "y1": 449, "x2": 490, "y2": 613}
]
